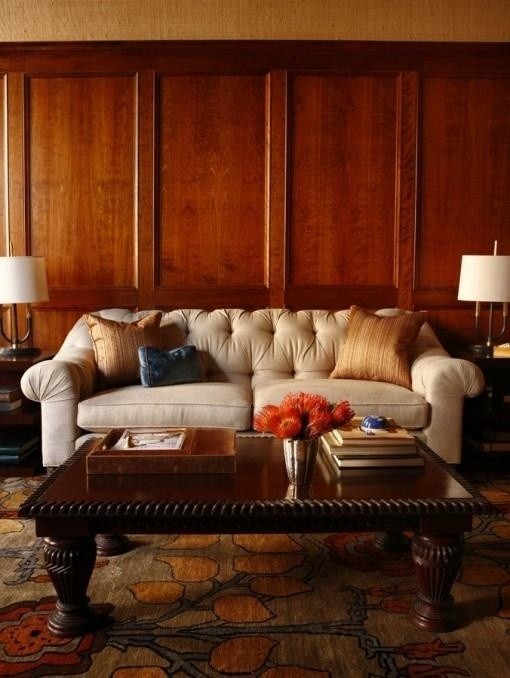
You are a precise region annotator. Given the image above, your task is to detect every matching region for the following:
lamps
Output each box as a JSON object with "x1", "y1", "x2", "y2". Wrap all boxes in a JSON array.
[
  {"x1": 454, "y1": 240, "x2": 509, "y2": 353},
  {"x1": 0, "y1": 239, "x2": 49, "y2": 357}
]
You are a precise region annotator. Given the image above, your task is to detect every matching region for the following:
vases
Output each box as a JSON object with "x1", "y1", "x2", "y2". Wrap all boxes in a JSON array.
[{"x1": 283, "y1": 433, "x2": 321, "y2": 486}]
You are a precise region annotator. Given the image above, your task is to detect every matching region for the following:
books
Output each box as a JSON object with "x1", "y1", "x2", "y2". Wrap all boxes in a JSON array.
[
  {"x1": 464, "y1": 428, "x2": 510, "y2": 452},
  {"x1": 0, "y1": 434, "x2": 40, "y2": 464},
  {"x1": 0, "y1": 385, "x2": 23, "y2": 412},
  {"x1": 321, "y1": 416, "x2": 425, "y2": 469}
]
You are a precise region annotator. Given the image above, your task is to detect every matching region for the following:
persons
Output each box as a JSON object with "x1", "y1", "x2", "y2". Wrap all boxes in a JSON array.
[{"x1": 127, "y1": 432, "x2": 180, "y2": 447}]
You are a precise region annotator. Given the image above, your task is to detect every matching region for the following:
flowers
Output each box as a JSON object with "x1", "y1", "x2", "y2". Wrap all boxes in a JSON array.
[{"x1": 249, "y1": 390, "x2": 356, "y2": 442}]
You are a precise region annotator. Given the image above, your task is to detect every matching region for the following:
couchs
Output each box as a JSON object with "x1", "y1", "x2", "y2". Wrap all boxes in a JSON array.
[{"x1": 20, "y1": 307, "x2": 486, "y2": 472}]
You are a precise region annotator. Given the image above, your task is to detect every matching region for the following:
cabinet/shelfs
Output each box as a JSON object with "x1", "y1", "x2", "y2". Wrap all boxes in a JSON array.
[
  {"x1": 0, "y1": 349, "x2": 60, "y2": 476},
  {"x1": 454, "y1": 348, "x2": 510, "y2": 481}
]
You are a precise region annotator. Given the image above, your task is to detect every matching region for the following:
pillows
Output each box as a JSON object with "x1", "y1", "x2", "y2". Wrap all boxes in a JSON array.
[
  {"x1": 81, "y1": 311, "x2": 163, "y2": 393},
  {"x1": 329, "y1": 305, "x2": 429, "y2": 392},
  {"x1": 135, "y1": 344, "x2": 201, "y2": 384}
]
